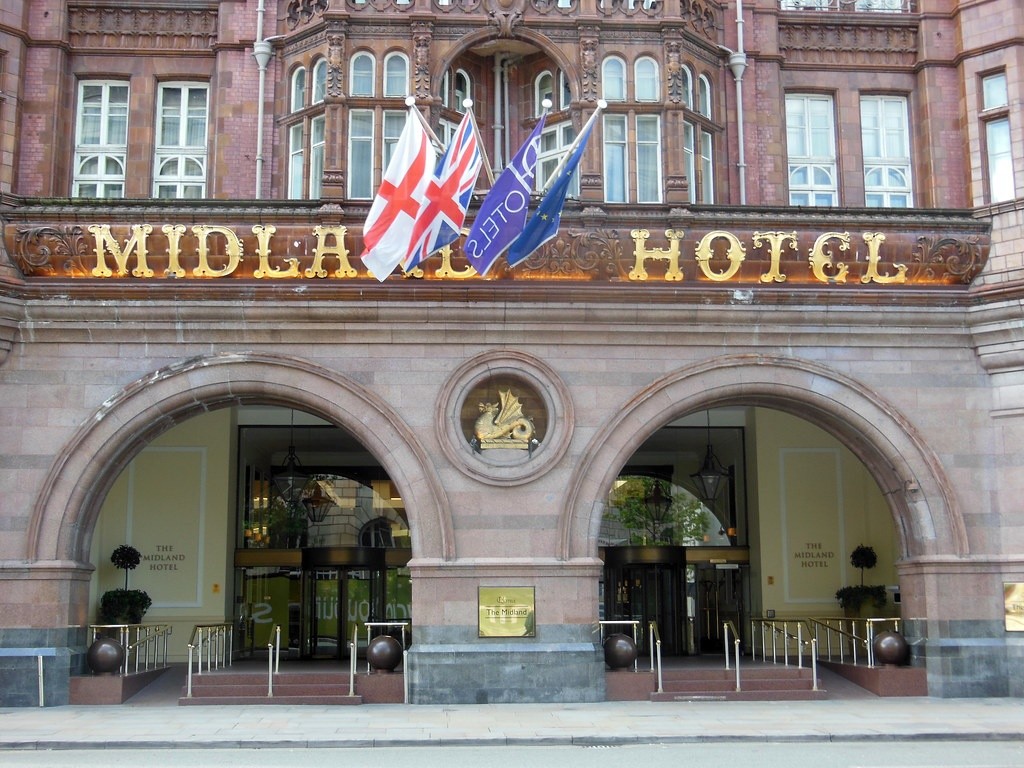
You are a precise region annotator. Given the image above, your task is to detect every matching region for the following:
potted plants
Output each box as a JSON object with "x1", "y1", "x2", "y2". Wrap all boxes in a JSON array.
[
  {"x1": 101, "y1": 543, "x2": 152, "y2": 672},
  {"x1": 836, "y1": 542, "x2": 886, "y2": 657}
]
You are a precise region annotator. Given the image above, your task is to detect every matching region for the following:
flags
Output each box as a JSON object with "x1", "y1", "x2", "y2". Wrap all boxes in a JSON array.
[
  {"x1": 359, "y1": 107, "x2": 437, "y2": 283},
  {"x1": 505, "y1": 114, "x2": 600, "y2": 269},
  {"x1": 462, "y1": 111, "x2": 548, "y2": 277},
  {"x1": 402, "y1": 111, "x2": 483, "y2": 274}
]
